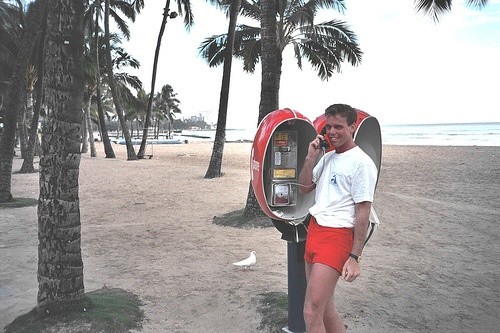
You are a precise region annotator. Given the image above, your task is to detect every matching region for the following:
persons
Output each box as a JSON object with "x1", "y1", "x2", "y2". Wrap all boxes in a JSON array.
[{"x1": 299, "y1": 104, "x2": 379, "y2": 333}]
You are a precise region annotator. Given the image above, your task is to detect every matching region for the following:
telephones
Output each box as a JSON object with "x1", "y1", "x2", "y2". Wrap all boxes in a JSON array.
[{"x1": 319, "y1": 123, "x2": 330, "y2": 150}]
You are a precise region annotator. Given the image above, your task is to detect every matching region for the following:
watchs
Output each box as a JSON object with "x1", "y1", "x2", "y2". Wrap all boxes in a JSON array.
[{"x1": 349, "y1": 253, "x2": 362, "y2": 263}]
